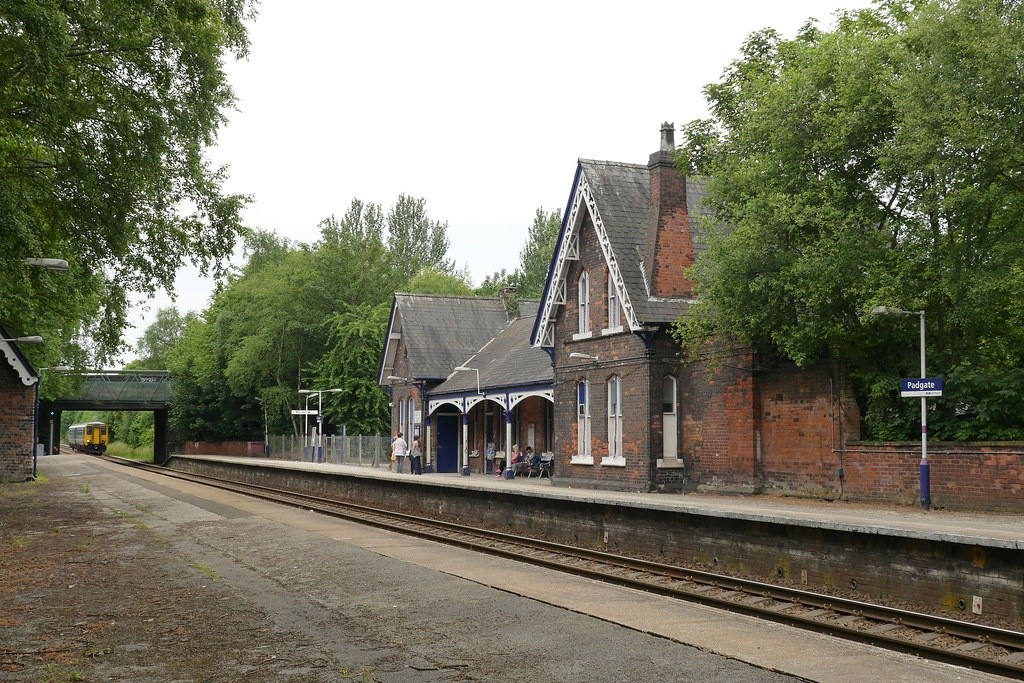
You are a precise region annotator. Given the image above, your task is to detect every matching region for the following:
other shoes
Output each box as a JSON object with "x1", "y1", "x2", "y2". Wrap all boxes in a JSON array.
[
  {"x1": 496, "y1": 469, "x2": 501, "y2": 473},
  {"x1": 495, "y1": 475, "x2": 502, "y2": 478}
]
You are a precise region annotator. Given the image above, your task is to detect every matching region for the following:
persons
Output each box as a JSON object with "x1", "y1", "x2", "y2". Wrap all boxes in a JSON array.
[
  {"x1": 391, "y1": 432, "x2": 407, "y2": 474},
  {"x1": 494, "y1": 444, "x2": 520, "y2": 478},
  {"x1": 512, "y1": 446, "x2": 535, "y2": 475},
  {"x1": 409, "y1": 435, "x2": 422, "y2": 475}
]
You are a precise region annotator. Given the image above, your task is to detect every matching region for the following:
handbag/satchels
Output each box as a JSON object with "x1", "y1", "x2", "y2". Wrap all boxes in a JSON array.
[{"x1": 390, "y1": 443, "x2": 397, "y2": 460}]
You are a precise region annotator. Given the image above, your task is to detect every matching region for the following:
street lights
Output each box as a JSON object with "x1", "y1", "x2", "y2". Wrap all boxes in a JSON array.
[
  {"x1": 304, "y1": 393, "x2": 318, "y2": 461},
  {"x1": 33, "y1": 365, "x2": 74, "y2": 479},
  {"x1": 872, "y1": 305, "x2": 930, "y2": 513},
  {"x1": 298, "y1": 388, "x2": 342, "y2": 463},
  {"x1": 255, "y1": 398, "x2": 270, "y2": 456}
]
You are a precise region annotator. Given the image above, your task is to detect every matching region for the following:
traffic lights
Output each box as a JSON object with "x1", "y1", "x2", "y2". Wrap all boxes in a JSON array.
[{"x1": 50, "y1": 411, "x2": 55, "y2": 417}]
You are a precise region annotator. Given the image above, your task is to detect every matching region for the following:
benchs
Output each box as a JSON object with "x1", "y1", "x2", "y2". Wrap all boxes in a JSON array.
[{"x1": 506, "y1": 453, "x2": 553, "y2": 478}]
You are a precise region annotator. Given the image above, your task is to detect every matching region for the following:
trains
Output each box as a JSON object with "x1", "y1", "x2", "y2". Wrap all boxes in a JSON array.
[{"x1": 68, "y1": 421, "x2": 109, "y2": 456}]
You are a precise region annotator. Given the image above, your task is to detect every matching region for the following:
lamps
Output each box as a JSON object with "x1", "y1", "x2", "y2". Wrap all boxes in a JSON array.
[
  {"x1": 389, "y1": 401, "x2": 396, "y2": 408},
  {"x1": 0, "y1": 335, "x2": 42, "y2": 344},
  {"x1": 37, "y1": 366, "x2": 75, "y2": 377},
  {"x1": 568, "y1": 352, "x2": 599, "y2": 360},
  {"x1": 454, "y1": 367, "x2": 485, "y2": 395},
  {"x1": 0, "y1": 257, "x2": 69, "y2": 269},
  {"x1": 386, "y1": 376, "x2": 409, "y2": 381}
]
